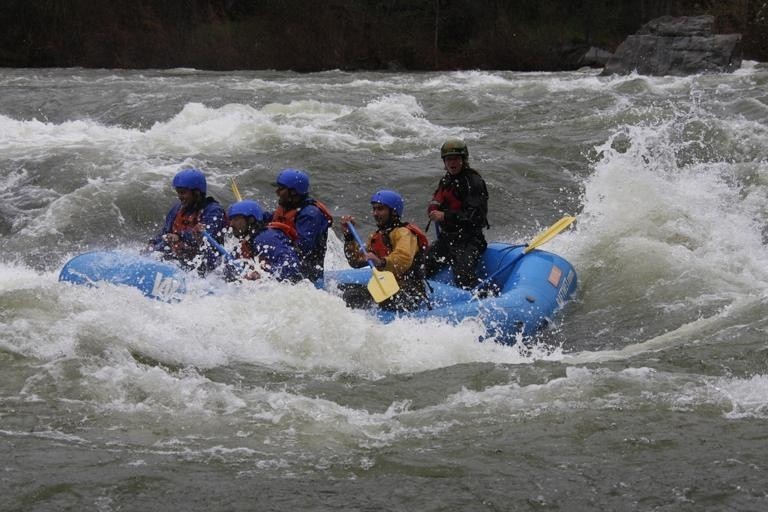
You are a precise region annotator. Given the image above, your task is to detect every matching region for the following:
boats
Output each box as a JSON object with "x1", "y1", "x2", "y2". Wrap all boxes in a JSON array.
[{"x1": 57, "y1": 238, "x2": 579, "y2": 348}]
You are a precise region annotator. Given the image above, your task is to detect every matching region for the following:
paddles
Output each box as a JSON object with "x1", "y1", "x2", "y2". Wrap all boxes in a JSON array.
[
  {"x1": 229, "y1": 175, "x2": 242, "y2": 203},
  {"x1": 346, "y1": 221, "x2": 400, "y2": 303},
  {"x1": 472, "y1": 217, "x2": 576, "y2": 291}
]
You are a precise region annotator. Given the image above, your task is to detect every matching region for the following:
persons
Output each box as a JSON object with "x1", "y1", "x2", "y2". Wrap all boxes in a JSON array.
[
  {"x1": 216, "y1": 200, "x2": 304, "y2": 285},
  {"x1": 271, "y1": 168, "x2": 333, "y2": 289},
  {"x1": 337, "y1": 190, "x2": 434, "y2": 312},
  {"x1": 140, "y1": 169, "x2": 227, "y2": 278},
  {"x1": 426, "y1": 140, "x2": 489, "y2": 290}
]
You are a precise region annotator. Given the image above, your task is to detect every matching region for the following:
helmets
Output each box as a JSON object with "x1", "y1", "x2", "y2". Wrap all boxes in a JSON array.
[
  {"x1": 441, "y1": 139, "x2": 469, "y2": 162},
  {"x1": 172, "y1": 169, "x2": 206, "y2": 195},
  {"x1": 228, "y1": 199, "x2": 264, "y2": 222},
  {"x1": 370, "y1": 190, "x2": 403, "y2": 218},
  {"x1": 277, "y1": 168, "x2": 309, "y2": 197}
]
